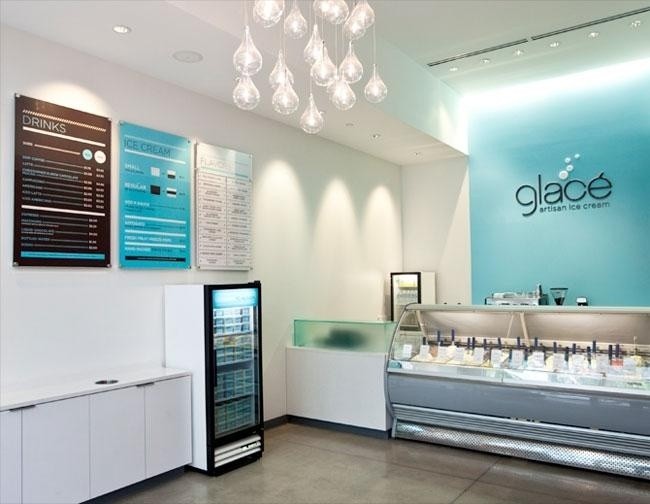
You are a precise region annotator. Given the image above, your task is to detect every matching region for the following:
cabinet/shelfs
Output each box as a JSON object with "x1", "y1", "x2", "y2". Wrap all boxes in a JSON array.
[
  {"x1": 286, "y1": 347, "x2": 393, "y2": 441},
  {"x1": 88, "y1": 370, "x2": 194, "y2": 500},
  {"x1": 0, "y1": 392, "x2": 89, "y2": 504}
]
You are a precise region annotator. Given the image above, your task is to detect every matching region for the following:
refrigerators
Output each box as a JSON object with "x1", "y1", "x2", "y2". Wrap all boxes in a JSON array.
[
  {"x1": 163, "y1": 281, "x2": 264, "y2": 478},
  {"x1": 384, "y1": 302, "x2": 649, "y2": 481}
]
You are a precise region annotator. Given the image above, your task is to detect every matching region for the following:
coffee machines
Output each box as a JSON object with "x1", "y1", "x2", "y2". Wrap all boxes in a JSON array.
[{"x1": 550, "y1": 287, "x2": 569, "y2": 305}]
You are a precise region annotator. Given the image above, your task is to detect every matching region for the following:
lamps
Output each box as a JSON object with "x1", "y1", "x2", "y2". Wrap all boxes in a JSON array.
[{"x1": 231, "y1": 0, "x2": 387, "y2": 134}]
